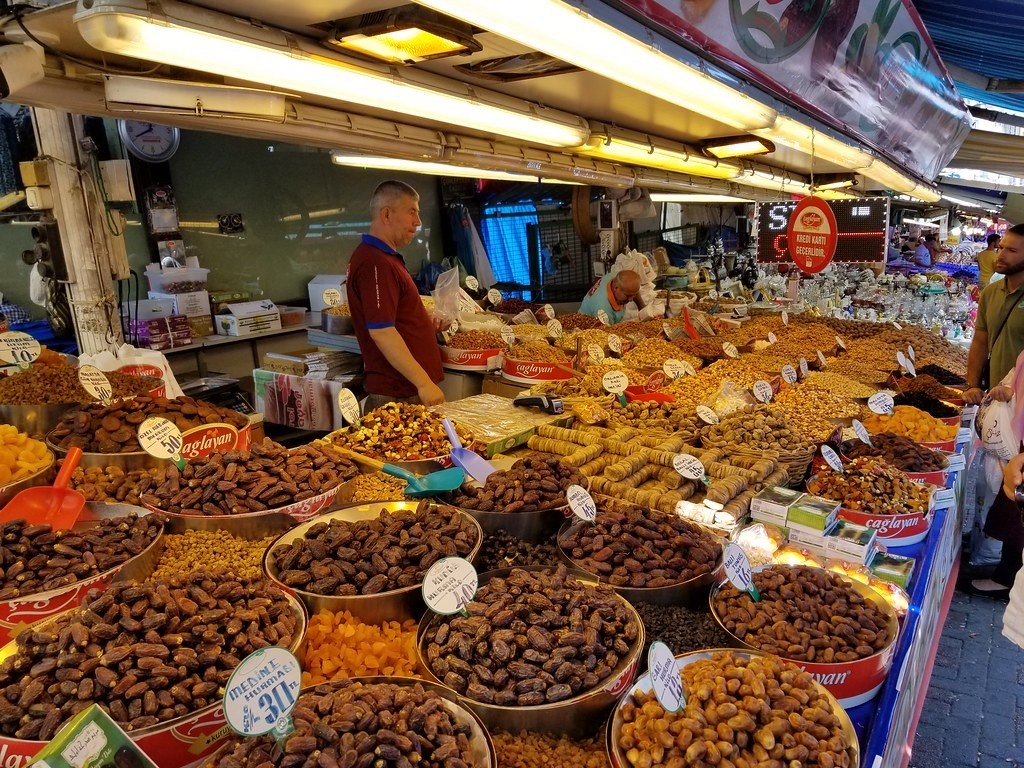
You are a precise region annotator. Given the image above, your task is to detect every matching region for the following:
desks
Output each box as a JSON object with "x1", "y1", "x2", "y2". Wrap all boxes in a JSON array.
[
  {"x1": 253, "y1": 368, "x2": 364, "y2": 431},
  {"x1": 161, "y1": 311, "x2": 322, "y2": 380}
]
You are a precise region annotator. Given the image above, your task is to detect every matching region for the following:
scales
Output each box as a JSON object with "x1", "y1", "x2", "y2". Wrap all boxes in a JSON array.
[{"x1": 178, "y1": 376, "x2": 256, "y2": 419}]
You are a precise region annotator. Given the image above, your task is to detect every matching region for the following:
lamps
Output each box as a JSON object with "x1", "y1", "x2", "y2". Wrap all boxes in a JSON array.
[{"x1": 0, "y1": 0, "x2": 956, "y2": 203}]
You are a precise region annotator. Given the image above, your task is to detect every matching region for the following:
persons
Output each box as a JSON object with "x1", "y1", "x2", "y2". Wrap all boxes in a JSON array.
[
  {"x1": 915, "y1": 234, "x2": 952, "y2": 273},
  {"x1": 346, "y1": 179, "x2": 445, "y2": 409},
  {"x1": 577, "y1": 270, "x2": 647, "y2": 324},
  {"x1": 977, "y1": 234, "x2": 1000, "y2": 285},
  {"x1": 961, "y1": 223, "x2": 1024, "y2": 406},
  {"x1": 955, "y1": 350, "x2": 1024, "y2": 603}
]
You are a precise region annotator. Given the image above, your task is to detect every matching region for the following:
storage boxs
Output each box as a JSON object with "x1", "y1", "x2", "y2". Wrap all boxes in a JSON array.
[
  {"x1": 458, "y1": 285, "x2": 484, "y2": 313},
  {"x1": 750, "y1": 484, "x2": 916, "y2": 589},
  {"x1": 307, "y1": 274, "x2": 347, "y2": 311},
  {"x1": 10, "y1": 319, "x2": 78, "y2": 357},
  {"x1": 120, "y1": 268, "x2": 308, "y2": 351},
  {"x1": 443, "y1": 371, "x2": 528, "y2": 401}
]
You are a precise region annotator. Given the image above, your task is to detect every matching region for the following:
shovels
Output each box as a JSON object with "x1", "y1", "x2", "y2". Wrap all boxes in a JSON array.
[
  {"x1": 683, "y1": 306, "x2": 700, "y2": 340},
  {"x1": 664, "y1": 289, "x2": 675, "y2": 319},
  {"x1": 0, "y1": 448, "x2": 85, "y2": 532},
  {"x1": 314, "y1": 438, "x2": 465, "y2": 497},
  {"x1": 441, "y1": 419, "x2": 497, "y2": 486},
  {"x1": 553, "y1": 363, "x2": 673, "y2": 404},
  {"x1": 706, "y1": 304, "x2": 718, "y2": 316}
]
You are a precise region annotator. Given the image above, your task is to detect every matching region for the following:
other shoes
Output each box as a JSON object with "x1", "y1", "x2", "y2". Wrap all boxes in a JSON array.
[{"x1": 972, "y1": 579, "x2": 1010, "y2": 590}]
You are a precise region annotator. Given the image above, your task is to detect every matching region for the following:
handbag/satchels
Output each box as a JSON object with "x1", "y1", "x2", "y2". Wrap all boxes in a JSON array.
[
  {"x1": 978, "y1": 358, "x2": 991, "y2": 390},
  {"x1": 974, "y1": 366, "x2": 1020, "y2": 498}
]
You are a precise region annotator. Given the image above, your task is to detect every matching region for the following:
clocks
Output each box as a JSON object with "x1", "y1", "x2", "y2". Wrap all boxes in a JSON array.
[{"x1": 117, "y1": 119, "x2": 179, "y2": 162}]
